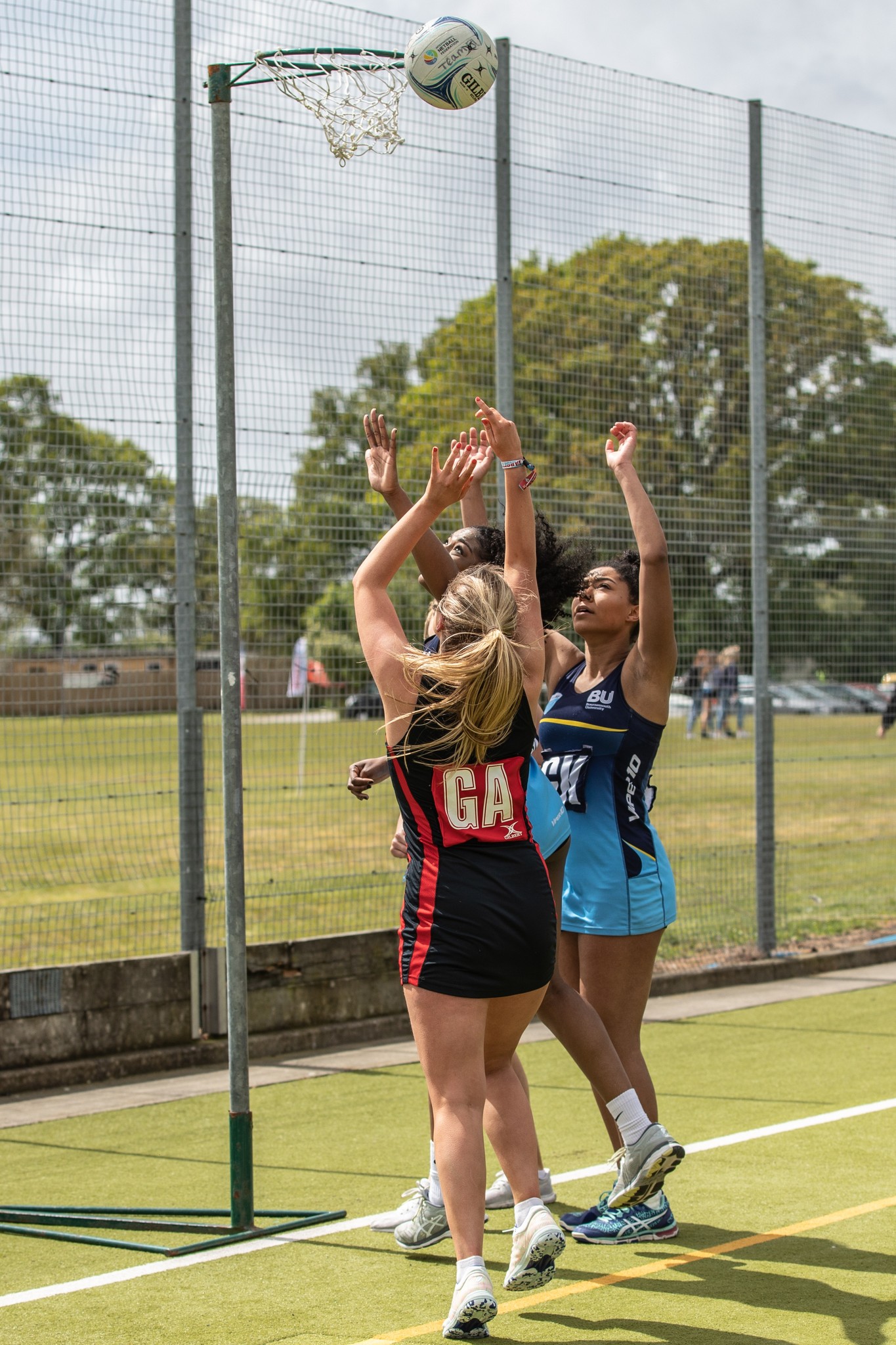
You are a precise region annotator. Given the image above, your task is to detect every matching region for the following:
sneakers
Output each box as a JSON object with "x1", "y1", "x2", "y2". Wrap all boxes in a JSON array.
[
  {"x1": 485, "y1": 1168, "x2": 557, "y2": 1210},
  {"x1": 441, "y1": 1266, "x2": 498, "y2": 1339},
  {"x1": 606, "y1": 1122, "x2": 685, "y2": 1209},
  {"x1": 393, "y1": 1181, "x2": 488, "y2": 1249},
  {"x1": 370, "y1": 1177, "x2": 430, "y2": 1232},
  {"x1": 559, "y1": 1180, "x2": 621, "y2": 1234},
  {"x1": 502, "y1": 1205, "x2": 566, "y2": 1292},
  {"x1": 571, "y1": 1189, "x2": 679, "y2": 1244}
]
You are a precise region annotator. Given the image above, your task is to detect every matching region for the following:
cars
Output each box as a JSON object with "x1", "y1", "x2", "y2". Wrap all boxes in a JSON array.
[
  {"x1": 341, "y1": 682, "x2": 386, "y2": 719},
  {"x1": 666, "y1": 675, "x2": 896, "y2": 717}
]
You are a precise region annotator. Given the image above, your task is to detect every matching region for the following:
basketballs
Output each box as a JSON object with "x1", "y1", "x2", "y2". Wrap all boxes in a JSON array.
[{"x1": 403, "y1": 16, "x2": 498, "y2": 111}]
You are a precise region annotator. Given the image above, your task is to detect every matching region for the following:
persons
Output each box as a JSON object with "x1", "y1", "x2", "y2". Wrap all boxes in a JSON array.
[
  {"x1": 352, "y1": 400, "x2": 679, "y2": 1337},
  {"x1": 683, "y1": 646, "x2": 750, "y2": 739},
  {"x1": 876, "y1": 683, "x2": 896, "y2": 739}
]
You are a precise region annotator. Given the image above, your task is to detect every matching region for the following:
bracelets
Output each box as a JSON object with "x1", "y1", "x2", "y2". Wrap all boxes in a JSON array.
[{"x1": 500, "y1": 455, "x2": 537, "y2": 491}]
